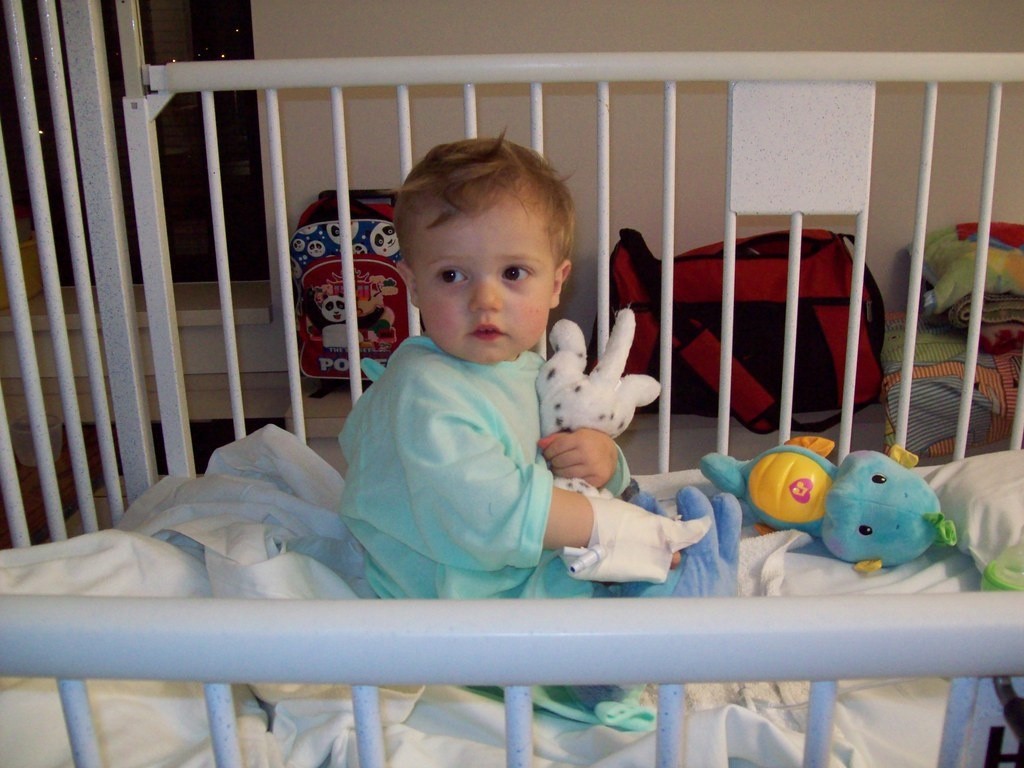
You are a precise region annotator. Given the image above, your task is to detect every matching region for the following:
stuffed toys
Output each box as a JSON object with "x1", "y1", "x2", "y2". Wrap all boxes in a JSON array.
[
  {"x1": 700, "y1": 437, "x2": 958, "y2": 571},
  {"x1": 537, "y1": 308, "x2": 662, "y2": 499},
  {"x1": 600, "y1": 487, "x2": 743, "y2": 597}
]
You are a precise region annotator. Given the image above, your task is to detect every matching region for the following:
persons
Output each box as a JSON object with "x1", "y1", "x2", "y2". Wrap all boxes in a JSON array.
[{"x1": 341, "y1": 133, "x2": 711, "y2": 599}]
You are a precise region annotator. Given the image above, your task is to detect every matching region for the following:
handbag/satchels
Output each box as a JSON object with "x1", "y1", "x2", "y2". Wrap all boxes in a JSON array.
[{"x1": 583, "y1": 227, "x2": 887, "y2": 432}]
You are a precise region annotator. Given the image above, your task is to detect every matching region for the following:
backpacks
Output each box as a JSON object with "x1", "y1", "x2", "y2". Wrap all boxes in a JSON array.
[{"x1": 288, "y1": 191, "x2": 426, "y2": 400}]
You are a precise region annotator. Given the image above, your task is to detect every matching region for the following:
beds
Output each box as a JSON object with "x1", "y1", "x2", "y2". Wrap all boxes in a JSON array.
[{"x1": 0, "y1": 1, "x2": 1024, "y2": 768}]
